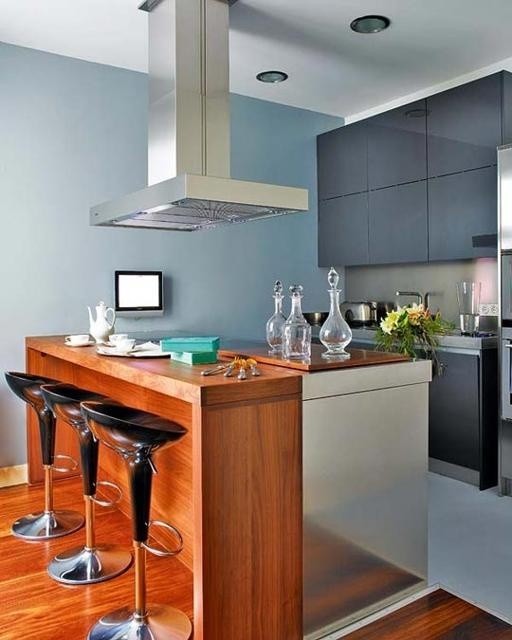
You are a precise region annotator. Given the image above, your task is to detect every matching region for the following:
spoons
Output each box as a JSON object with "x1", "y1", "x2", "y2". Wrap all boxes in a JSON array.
[{"x1": 237, "y1": 358, "x2": 260, "y2": 380}]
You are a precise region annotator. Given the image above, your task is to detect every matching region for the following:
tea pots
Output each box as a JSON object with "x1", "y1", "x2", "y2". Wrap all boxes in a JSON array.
[{"x1": 87, "y1": 301, "x2": 116, "y2": 344}]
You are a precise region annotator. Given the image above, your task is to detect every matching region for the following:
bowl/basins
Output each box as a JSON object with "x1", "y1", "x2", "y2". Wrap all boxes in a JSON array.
[{"x1": 302, "y1": 312, "x2": 329, "y2": 326}]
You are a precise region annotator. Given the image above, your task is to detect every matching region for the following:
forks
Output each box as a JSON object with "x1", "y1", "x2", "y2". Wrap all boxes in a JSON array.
[{"x1": 201, "y1": 355, "x2": 242, "y2": 377}]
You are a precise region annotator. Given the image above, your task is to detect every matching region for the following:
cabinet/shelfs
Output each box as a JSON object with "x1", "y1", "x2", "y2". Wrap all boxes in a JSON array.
[{"x1": 315, "y1": 68, "x2": 509, "y2": 272}]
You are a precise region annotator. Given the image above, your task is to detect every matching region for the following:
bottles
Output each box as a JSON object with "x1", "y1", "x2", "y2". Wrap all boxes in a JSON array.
[
  {"x1": 319, "y1": 266, "x2": 353, "y2": 360},
  {"x1": 266, "y1": 280, "x2": 287, "y2": 353},
  {"x1": 282, "y1": 284, "x2": 312, "y2": 360}
]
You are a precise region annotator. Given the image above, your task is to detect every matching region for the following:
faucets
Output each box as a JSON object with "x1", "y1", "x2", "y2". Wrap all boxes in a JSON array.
[
  {"x1": 425, "y1": 290, "x2": 444, "y2": 308},
  {"x1": 396, "y1": 291, "x2": 423, "y2": 306}
]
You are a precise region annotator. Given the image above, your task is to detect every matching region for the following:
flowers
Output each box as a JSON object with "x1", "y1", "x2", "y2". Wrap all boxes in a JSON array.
[{"x1": 373, "y1": 302, "x2": 457, "y2": 380}]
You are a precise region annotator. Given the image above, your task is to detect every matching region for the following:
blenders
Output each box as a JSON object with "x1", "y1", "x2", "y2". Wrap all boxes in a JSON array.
[{"x1": 455, "y1": 279, "x2": 483, "y2": 337}]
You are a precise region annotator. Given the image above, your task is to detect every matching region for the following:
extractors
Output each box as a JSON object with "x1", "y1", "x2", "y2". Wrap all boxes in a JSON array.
[{"x1": 89, "y1": 0, "x2": 309, "y2": 233}]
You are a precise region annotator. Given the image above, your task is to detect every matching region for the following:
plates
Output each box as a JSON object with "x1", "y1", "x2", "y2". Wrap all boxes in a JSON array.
[
  {"x1": 96, "y1": 343, "x2": 171, "y2": 358},
  {"x1": 64, "y1": 341, "x2": 95, "y2": 347}
]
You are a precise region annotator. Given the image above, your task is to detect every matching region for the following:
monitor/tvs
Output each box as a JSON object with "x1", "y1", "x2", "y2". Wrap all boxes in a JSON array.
[{"x1": 114, "y1": 268, "x2": 164, "y2": 317}]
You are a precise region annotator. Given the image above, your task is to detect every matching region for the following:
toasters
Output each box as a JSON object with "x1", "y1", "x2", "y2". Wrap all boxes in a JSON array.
[{"x1": 339, "y1": 301, "x2": 376, "y2": 328}]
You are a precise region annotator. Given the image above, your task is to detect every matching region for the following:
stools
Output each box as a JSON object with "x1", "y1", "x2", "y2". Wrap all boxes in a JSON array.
[
  {"x1": 80, "y1": 401, "x2": 193, "y2": 640},
  {"x1": 38, "y1": 384, "x2": 133, "y2": 586},
  {"x1": 1, "y1": 370, "x2": 86, "y2": 542}
]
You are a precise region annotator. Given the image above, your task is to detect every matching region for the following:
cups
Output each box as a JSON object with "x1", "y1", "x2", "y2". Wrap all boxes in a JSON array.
[
  {"x1": 116, "y1": 338, "x2": 136, "y2": 349},
  {"x1": 64, "y1": 335, "x2": 90, "y2": 344},
  {"x1": 108, "y1": 333, "x2": 128, "y2": 346}
]
